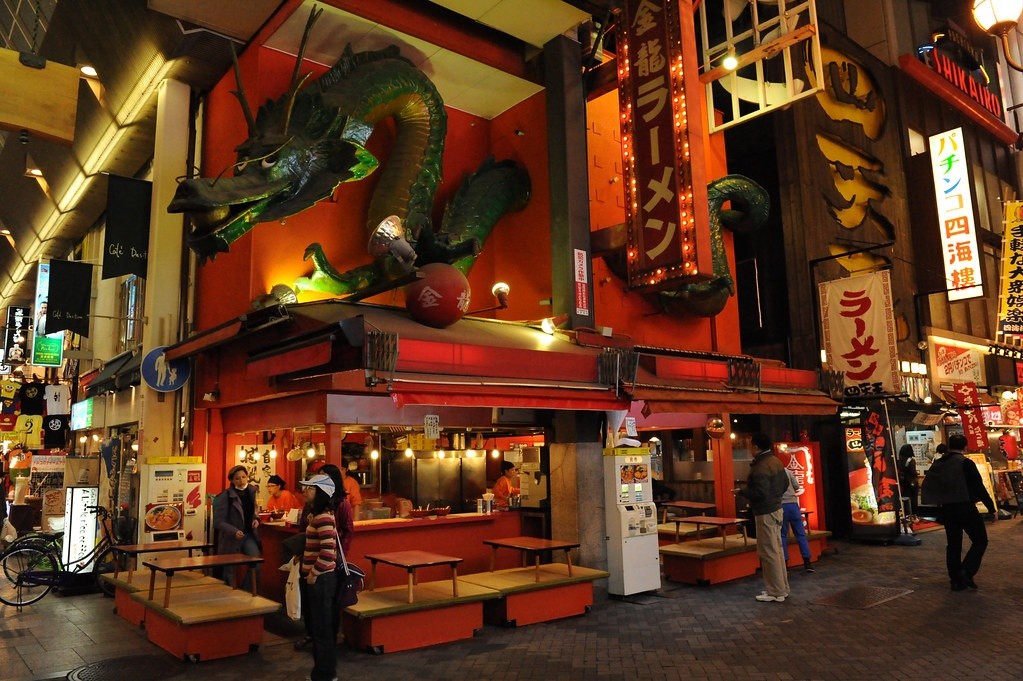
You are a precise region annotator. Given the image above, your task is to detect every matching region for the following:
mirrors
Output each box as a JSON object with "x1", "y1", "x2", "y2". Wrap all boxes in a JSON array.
[{"x1": 706, "y1": 417, "x2": 726, "y2": 441}]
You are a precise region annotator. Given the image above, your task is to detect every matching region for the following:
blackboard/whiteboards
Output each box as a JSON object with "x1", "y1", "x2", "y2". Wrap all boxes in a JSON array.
[{"x1": 1008, "y1": 471, "x2": 1023, "y2": 516}]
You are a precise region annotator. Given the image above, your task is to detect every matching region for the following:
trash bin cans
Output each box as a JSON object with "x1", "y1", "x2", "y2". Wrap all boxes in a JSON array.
[{"x1": 9, "y1": 505, "x2": 42, "y2": 538}]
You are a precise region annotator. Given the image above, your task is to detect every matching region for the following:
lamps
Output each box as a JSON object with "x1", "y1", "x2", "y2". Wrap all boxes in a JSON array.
[
  {"x1": 253, "y1": 282, "x2": 299, "y2": 312},
  {"x1": 530, "y1": 313, "x2": 569, "y2": 336},
  {"x1": 464, "y1": 283, "x2": 512, "y2": 316},
  {"x1": 0, "y1": 222, "x2": 28, "y2": 265},
  {"x1": 75, "y1": 61, "x2": 123, "y2": 128},
  {"x1": 25, "y1": 152, "x2": 62, "y2": 214},
  {"x1": 366, "y1": 216, "x2": 422, "y2": 268},
  {"x1": 203, "y1": 393, "x2": 216, "y2": 401}
]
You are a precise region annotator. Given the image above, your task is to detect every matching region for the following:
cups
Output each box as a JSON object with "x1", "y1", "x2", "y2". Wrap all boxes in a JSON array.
[{"x1": 482, "y1": 500, "x2": 493, "y2": 514}]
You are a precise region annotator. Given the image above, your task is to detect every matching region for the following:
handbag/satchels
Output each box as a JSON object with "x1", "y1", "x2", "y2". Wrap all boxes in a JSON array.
[
  {"x1": 0, "y1": 516, "x2": 17, "y2": 545},
  {"x1": 739, "y1": 515, "x2": 757, "y2": 537},
  {"x1": 334, "y1": 561, "x2": 366, "y2": 609},
  {"x1": 277, "y1": 555, "x2": 300, "y2": 622}
]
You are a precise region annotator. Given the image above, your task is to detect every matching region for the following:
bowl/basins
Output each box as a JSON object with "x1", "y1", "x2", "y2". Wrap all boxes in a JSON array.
[
  {"x1": 621, "y1": 465, "x2": 647, "y2": 483},
  {"x1": 258, "y1": 513, "x2": 271, "y2": 522},
  {"x1": 271, "y1": 512, "x2": 285, "y2": 520},
  {"x1": 145, "y1": 505, "x2": 181, "y2": 530},
  {"x1": 409, "y1": 508, "x2": 451, "y2": 518}
]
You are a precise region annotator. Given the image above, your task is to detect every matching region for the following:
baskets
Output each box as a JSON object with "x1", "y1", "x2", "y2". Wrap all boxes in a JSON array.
[{"x1": 111, "y1": 517, "x2": 136, "y2": 542}]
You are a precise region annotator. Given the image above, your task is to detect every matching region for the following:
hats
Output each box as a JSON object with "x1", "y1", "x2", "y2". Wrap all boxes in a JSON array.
[{"x1": 299, "y1": 474, "x2": 334, "y2": 498}]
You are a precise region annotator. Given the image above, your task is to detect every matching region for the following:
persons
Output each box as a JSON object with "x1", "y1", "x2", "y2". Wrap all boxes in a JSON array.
[
  {"x1": 341, "y1": 457, "x2": 362, "y2": 522},
  {"x1": 491, "y1": 461, "x2": 520, "y2": 506},
  {"x1": 265, "y1": 474, "x2": 304, "y2": 512},
  {"x1": 730, "y1": 429, "x2": 790, "y2": 602},
  {"x1": 213, "y1": 465, "x2": 264, "y2": 587},
  {"x1": 0, "y1": 452, "x2": 9, "y2": 559},
  {"x1": 932, "y1": 443, "x2": 944, "y2": 524},
  {"x1": 781, "y1": 466, "x2": 816, "y2": 573},
  {"x1": 295, "y1": 464, "x2": 354, "y2": 681},
  {"x1": 898, "y1": 444, "x2": 920, "y2": 523},
  {"x1": 921, "y1": 434, "x2": 998, "y2": 591}
]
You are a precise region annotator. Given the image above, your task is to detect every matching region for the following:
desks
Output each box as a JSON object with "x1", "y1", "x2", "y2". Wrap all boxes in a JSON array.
[
  {"x1": 661, "y1": 500, "x2": 718, "y2": 525},
  {"x1": 673, "y1": 515, "x2": 749, "y2": 553},
  {"x1": 482, "y1": 536, "x2": 582, "y2": 582},
  {"x1": 786, "y1": 509, "x2": 815, "y2": 537},
  {"x1": 364, "y1": 550, "x2": 464, "y2": 604}
]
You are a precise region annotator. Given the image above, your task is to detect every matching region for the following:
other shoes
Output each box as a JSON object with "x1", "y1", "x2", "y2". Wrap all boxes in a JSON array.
[
  {"x1": 804, "y1": 561, "x2": 815, "y2": 572},
  {"x1": 756, "y1": 588, "x2": 788, "y2": 601},
  {"x1": 959, "y1": 570, "x2": 978, "y2": 588},
  {"x1": 951, "y1": 581, "x2": 963, "y2": 590},
  {"x1": 306, "y1": 673, "x2": 337, "y2": 681},
  {"x1": 294, "y1": 634, "x2": 314, "y2": 649}
]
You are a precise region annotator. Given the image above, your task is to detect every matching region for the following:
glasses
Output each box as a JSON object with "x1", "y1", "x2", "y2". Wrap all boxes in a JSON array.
[{"x1": 302, "y1": 485, "x2": 315, "y2": 491}]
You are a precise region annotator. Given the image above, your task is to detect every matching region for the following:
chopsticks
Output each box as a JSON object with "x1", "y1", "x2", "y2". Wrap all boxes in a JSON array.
[{"x1": 482, "y1": 493, "x2": 494, "y2": 500}]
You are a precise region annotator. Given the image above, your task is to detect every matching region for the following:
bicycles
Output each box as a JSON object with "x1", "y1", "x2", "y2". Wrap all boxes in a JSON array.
[{"x1": 0, "y1": 506, "x2": 135, "y2": 612}]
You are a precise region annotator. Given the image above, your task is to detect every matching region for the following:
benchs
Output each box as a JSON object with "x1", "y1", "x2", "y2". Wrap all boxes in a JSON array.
[
  {"x1": 142, "y1": 552, "x2": 265, "y2": 607},
  {"x1": 111, "y1": 540, "x2": 216, "y2": 582}
]
